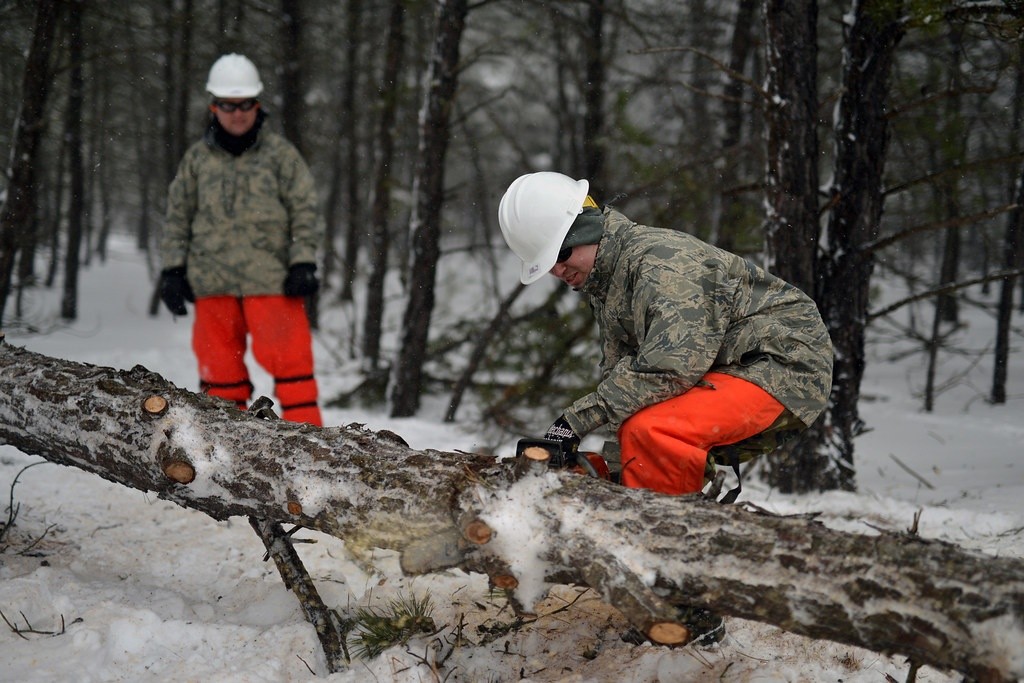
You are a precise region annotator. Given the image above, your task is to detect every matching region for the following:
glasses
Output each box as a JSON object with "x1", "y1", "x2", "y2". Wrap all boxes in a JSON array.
[
  {"x1": 557, "y1": 247, "x2": 573, "y2": 263},
  {"x1": 216, "y1": 98, "x2": 257, "y2": 112}
]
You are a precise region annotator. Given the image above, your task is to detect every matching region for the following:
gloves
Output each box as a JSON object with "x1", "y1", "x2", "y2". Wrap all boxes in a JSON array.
[
  {"x1": 159, "y1": 264, "x2": 195, "y2": 315},
  {"x1": 545, "y1": 413, "x2": 581, "y2": 468},
  {"x1": 283, "y1": 263, "x2": 317, "y2": 298}
]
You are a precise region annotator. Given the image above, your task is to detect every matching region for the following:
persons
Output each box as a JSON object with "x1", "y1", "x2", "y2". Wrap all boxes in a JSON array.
[
  {"x1": 498, "y1": 172, "x2": 832, "y2": 647},
  {"x1": 159, "y1": 54, "x2": 323, "y2": 428}
]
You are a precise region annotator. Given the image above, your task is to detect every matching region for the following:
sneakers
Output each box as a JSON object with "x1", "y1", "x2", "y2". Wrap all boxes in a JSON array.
[{"x1": 583, "y1": 608, "x2": 725, "y2": 660}]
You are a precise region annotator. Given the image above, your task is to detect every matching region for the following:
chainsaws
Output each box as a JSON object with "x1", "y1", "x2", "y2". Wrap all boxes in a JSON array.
[{"x1": 400, "y1": 435, "x2": 725, "y2": 580}]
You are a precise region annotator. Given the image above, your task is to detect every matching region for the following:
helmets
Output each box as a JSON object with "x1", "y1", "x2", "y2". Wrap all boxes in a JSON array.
[
  {"x1": 207, "y1": 54, "x2": 262, "y2": 97},
  {"x1": 498, "y1": 171, "x2": 590, "y2": 284}
]
